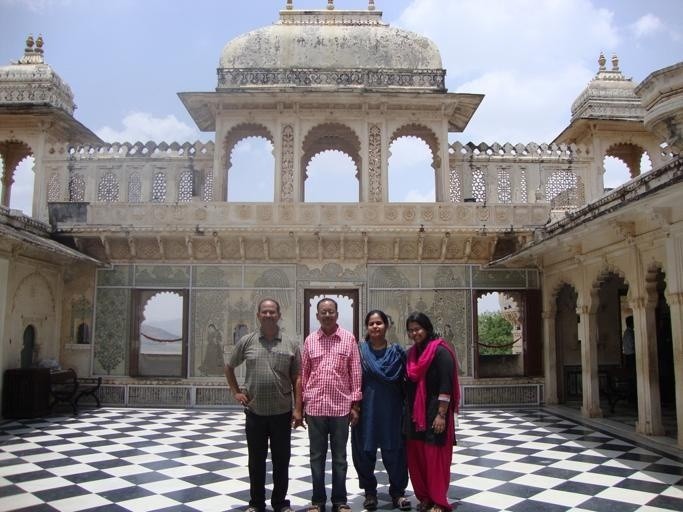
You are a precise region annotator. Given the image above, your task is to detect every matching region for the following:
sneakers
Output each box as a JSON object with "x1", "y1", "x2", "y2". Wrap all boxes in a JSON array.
[
  {"x1": 392, "y1": 496, "x2": 412, "y2": 509},
  {"x1": 307, "y1": 504, "x2": 324, "y2": 512},
  {"x1": 363, "y1": 494, "x2": 378, "y2": 509},
  {"x1": 278, "y1": 505, "x2": 296, "y2": 512},
  {"x1": 416, "y1": 502, "x2": 444, "y2": 512},
  {"x1": 336, "y1": 503, "x2": 352, "y2": 512},
  {"x1": 244, "y1": 505, "x2": 264, "y2": 512}
]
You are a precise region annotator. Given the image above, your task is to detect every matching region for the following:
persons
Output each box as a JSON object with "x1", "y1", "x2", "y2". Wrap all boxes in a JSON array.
[
  {"x1": 401, "y1": 311, "x2": 461, "y2": 512},
  {"x1": 224, "y1": 299, "x2": 301, "y2": 511},
  {"x1": 622, "y1": 315, "x2": 636, "y2": 404},
  {"x1": 294, "y1": 298, "x2": 364, "y2": 512},
  {"x1": 350, "y1": 309, "x2": 413, "y2": 512}
]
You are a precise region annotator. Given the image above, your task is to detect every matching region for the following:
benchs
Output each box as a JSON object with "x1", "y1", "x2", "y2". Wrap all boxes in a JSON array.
[{"x1": 48, "y1": 368, "x2": 102, "y2": 415}]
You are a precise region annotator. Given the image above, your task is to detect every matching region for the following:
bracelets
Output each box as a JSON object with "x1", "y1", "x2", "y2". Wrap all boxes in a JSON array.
[
  {"x1": 353, "y1": 406, "x2": 361, "y2": 412},
  {"x1": 438, "y1": 412, "x2": 448, "y2": 419}
]
